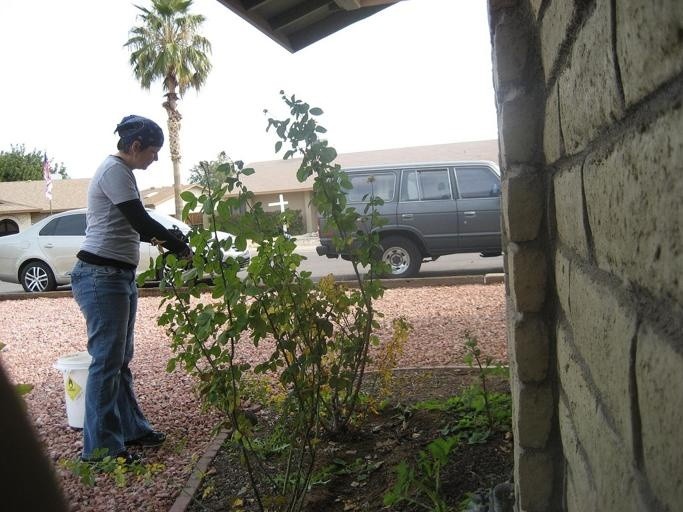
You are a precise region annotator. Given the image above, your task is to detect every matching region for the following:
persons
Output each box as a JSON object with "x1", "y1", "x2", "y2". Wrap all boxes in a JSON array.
[{"x1": 70, "y1": 114, "x2": 194, "y2": 467}]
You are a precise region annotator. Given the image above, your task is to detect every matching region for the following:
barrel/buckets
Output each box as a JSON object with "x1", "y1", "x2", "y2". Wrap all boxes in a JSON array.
[{"x1": 56, "y1": 353, "x2": 93, "y2": 430}]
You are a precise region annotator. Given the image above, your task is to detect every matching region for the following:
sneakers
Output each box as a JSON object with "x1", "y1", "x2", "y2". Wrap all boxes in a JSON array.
[
  {"x1": 124, "y1": 429, "x2": 166, "y2": 448},
  {"x1": 81, "y1": 450, "x2": 132, "y2": 464}
]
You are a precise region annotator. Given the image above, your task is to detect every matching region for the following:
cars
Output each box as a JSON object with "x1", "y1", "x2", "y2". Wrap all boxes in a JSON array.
[{"x1": 0, "y1": 207, "x2": 251, "y2": 292}]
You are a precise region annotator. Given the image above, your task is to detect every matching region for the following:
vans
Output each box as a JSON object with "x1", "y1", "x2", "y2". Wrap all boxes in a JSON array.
[{"x1": 316, "y1": 160, "x2": 501, "y2": 279}]
